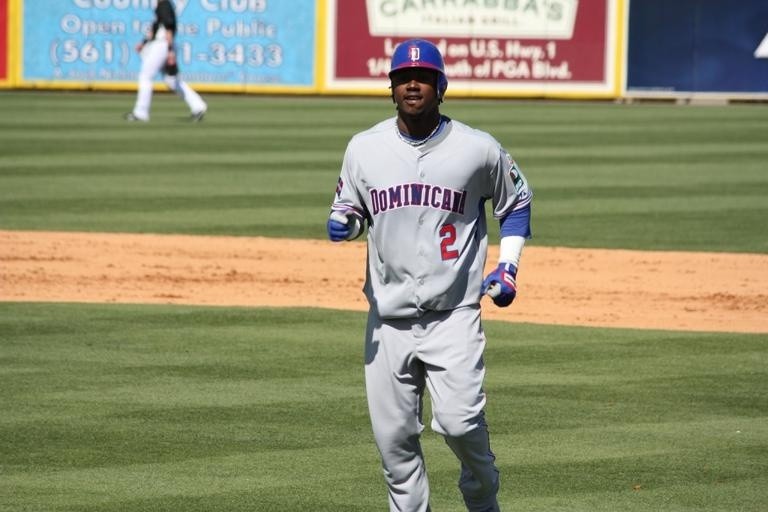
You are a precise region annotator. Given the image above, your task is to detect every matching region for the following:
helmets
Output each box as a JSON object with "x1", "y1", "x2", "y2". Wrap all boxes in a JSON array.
[{"x1": 389, "y1": 39, "x2": 447, "y2": 103}]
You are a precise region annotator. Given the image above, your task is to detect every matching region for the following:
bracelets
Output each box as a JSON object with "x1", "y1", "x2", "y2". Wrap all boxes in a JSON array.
[
  {"x1": 168, "y1": 45, "x2": 174, "y2": 52},
  {"x1": 141, "y1": 38, "x2": 148, "y2": 43}
]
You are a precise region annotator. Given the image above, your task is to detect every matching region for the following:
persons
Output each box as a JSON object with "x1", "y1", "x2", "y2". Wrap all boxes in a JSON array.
[
  {"x1": 124, "y1": 1, "x2": 208, "y2": 127},
  {"x1": 324, "y1": 38, "x2": 535, "y2": 511}
]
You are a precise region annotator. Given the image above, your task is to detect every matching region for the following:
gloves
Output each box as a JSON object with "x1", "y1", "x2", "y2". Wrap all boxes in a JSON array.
[
  {"x1": 326, "y1": 210, "x2": 361, "y2": 241},
  {"x1": 481, "y1": 262, "x2": 517, "y2": 307}
]
